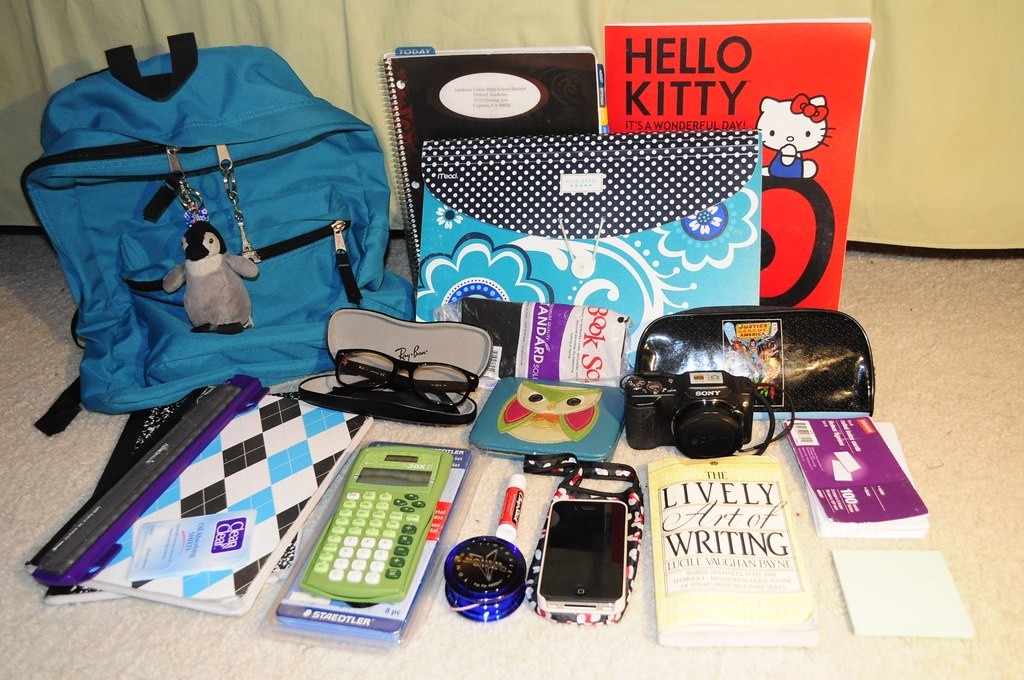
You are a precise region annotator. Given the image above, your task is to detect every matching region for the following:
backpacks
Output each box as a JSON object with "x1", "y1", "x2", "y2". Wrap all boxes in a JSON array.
[{"x1": 18, "y1": 29, "x2": 418, "y2": 439}]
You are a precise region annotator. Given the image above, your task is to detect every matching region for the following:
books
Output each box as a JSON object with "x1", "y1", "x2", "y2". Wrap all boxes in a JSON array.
[
  {"x1": 831, "y1": 547, "x2": 976, "y2": 640},
  {"x1": 781, "y1": 417, "x2": 928, "y2": 539},
  {"x1": 603, "y1": 18, "x2": 875, "y2": 309},
  {"x1": 43, "y1": 391, "x2": 375, "y2": 615},
  {"x1": 380, "y1": 46, "x2": 601, "y2": 286},
  {"x1": 647, "y1": 456, "x2": 819, "y2": 647}
]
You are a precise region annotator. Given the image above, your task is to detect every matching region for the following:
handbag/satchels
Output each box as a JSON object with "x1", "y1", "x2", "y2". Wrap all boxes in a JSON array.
[{"x1": 522, "y1": 451, "x2": 645, "y2": 630}]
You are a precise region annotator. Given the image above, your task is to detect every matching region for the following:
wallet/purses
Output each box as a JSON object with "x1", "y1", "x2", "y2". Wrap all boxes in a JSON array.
[{"x1": 468, "y1": 375, "x2": 627, "y2": 463}]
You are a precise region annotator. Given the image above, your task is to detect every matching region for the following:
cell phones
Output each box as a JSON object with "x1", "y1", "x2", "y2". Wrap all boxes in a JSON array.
[{"x1": 537, "y1": 498, "x2": 628, "y2": 614}]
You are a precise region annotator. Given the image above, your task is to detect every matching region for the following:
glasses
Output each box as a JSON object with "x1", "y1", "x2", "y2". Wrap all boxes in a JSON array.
[{"x1": 325, "y1": 348, "x2": 480, "y2": 415}]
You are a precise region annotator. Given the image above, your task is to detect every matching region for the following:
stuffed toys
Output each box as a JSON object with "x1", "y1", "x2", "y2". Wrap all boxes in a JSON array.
[{"x1": 163, "y1": 222, "x2": 259, "y2": 335}]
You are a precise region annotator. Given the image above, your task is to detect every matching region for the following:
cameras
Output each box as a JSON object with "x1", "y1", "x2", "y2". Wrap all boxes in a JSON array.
[{"x1": 624, "y1": 370, "x2": 754, "y2": 459}]
